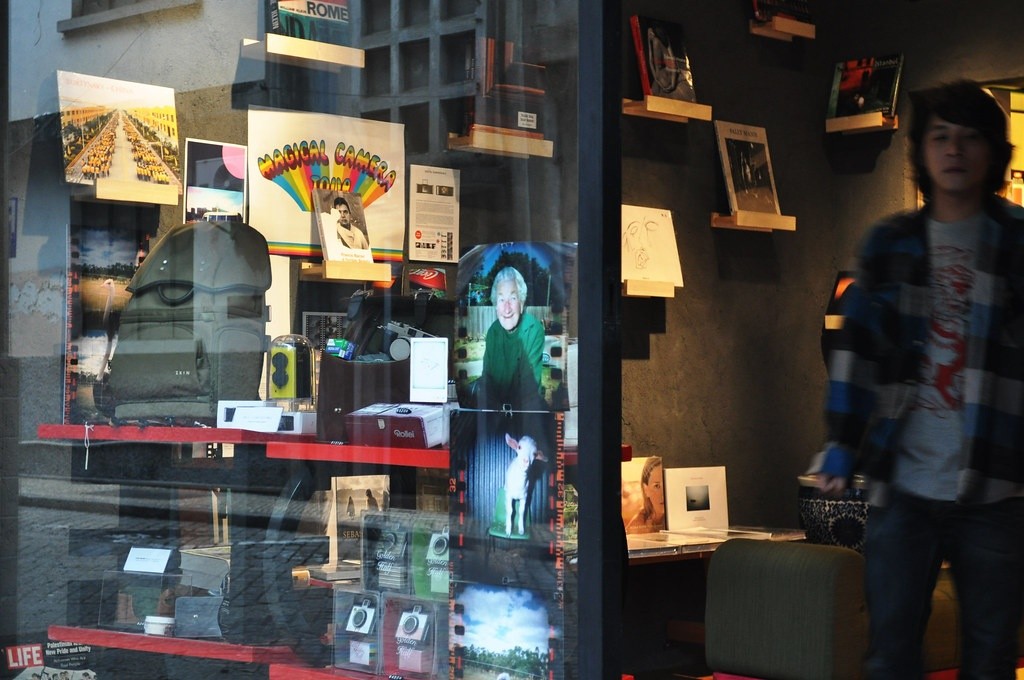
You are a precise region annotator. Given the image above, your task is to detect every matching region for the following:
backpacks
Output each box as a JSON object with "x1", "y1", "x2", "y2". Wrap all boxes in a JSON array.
[
  {"x1": 107, "y1": 221, "x2": 272, "y2": 418},
  {"x1": 343, "y1": 290, "x2": 455, "y2": 357}
]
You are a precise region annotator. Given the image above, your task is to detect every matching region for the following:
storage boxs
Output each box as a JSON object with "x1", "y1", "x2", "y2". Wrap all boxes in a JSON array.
[
  {"x1": 329, "y1": 585, "x2": 380, "y2": 675},
  {"x1": 97, "y1": 570, "x2": 192, "y2": 633},
  {"x1": 361, "y1": 510, "x2": 449, "y2": 601},
  {"x1": 380, "y1": 591, "x2": 448, "y2": 679}
]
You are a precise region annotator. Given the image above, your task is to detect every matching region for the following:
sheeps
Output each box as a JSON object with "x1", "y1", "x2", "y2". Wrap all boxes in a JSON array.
[{"x1": 505, "y1": 433, "x2": 549, "y2": 535}]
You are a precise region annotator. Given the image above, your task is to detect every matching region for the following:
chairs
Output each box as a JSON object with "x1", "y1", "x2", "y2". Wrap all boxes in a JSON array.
[{"x1": 706, "y1": 536, "x2": 873, "y2": 679}]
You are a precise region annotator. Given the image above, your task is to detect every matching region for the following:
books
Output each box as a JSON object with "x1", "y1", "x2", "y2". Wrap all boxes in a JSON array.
[
  {"x1": 753, "y1": 0, "x2": 812, "y2": 24},
  {"x1": 264, "y1": 0, "x2": 353, "y2": 47},
  {"x1": 307, "y1": 563, "x2": 361, "y2": 580},
  {"x1": 714, "y1": 119, "x2": 781, "y2": 218},
  {"x1": 826, "y1": 50, "x2": 905, "y2": 119},
  {"x1": 312, "y1": 190, "x2": 374, "y2": 261},
  {"x1": 327, "y1": 475, "x2": 389, "y2": 581},
  {"x1": 630, "y1": 14, "x2": 696, "y2": 104}
]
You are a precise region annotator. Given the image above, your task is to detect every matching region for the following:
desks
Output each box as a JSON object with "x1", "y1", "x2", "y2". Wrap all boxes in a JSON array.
[{"x1": 627, "y1": 528, "x2": 809, "y2": 566}]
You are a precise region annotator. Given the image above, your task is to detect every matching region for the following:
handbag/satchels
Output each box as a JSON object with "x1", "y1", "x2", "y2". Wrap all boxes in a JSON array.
[
  {"x1": 217, "y1": 536, "x2": 333, "y2": 643},
  {"x1": 174, "y1": 596, "x2": 224, "y2": 637}
]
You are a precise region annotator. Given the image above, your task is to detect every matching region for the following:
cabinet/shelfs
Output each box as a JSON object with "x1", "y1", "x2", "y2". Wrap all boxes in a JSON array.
[
  {"x1": 38, "y1": 424, "x2": 313, "y2": 663},
  {"x1": 268, "y1": 437, "x2": 454, "y2": 680}
]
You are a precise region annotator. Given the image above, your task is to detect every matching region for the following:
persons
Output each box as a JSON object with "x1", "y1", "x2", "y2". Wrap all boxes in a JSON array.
[
  {"x1": 835, "y1": 56, "x2": 883, "y2": 117},
  {"x1": 333, "y1": 197, "x2": 368, "y2": 249},
  {"x1": 366, "y1": 489, "x2": 378, "y2": 511},
  {"x1": 454, "y1": 267, "x2": 569, "y2": 454},
  {"x1": 625, "y1": 457, "x2": 665, "y2": 534},
  {"x1": 823, "y1": 77, "x2": 1024, "y2": 680},
  {"x1": 347, "y1": 495, "x2": 354, "y2": 518}
]
